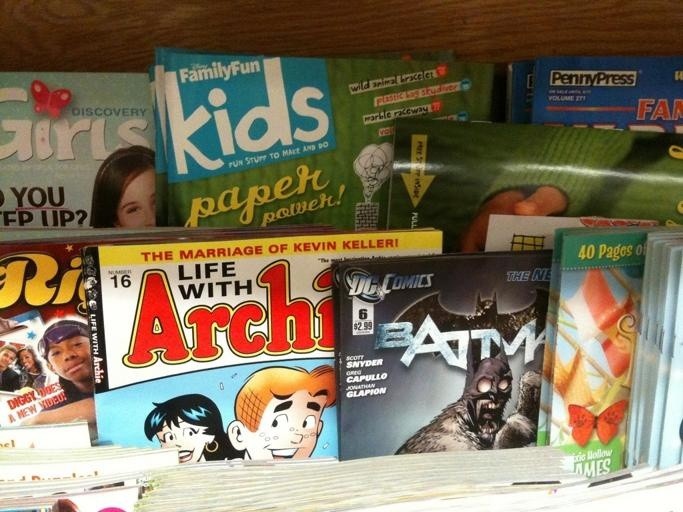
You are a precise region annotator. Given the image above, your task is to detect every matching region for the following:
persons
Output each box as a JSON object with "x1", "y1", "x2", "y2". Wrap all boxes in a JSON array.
[
  {"x1": 0, "y1": 343, "x2": 19, "y2": 395},
  {"x1": 20, "y1": 318, "x2": 93, "y2": 427},
  {"x1": 13, "y1": 346, "x2": 48, "y2": 389},
  {"x1": 88, "y1": 145, "x2": 157, "y2": 227}
]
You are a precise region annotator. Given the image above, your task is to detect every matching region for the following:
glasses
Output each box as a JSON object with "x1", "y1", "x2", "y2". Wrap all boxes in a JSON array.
[{"x1": 37, "y1": 324, "x2": 90, "y2": 358}]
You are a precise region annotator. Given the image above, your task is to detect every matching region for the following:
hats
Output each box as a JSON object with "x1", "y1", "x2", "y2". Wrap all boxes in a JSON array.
[{"x1": 45, "y1": 320, "x2": 91, "y2": 334}]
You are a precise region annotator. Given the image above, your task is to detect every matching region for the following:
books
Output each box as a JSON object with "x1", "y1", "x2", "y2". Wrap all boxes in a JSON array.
[
  {"x1": 486, "y1": 217, "x2": 683, "y2": 477},
  {"x1": 81, "y1": 228, "x2": 443, "y2": 459},
  {"x1": 387, "y1": 116, "x2": 681, "y2": 252},
  {"x1": 0, "y1": 236, "x2": 163, "y2": 430},
  {"x1": 491, "y1": 58, "x2": 682, "y2": 134},
  {"x1": 150, "y1": 54, "x2": 493, "y2": 231},
  {"x1": 335, "y1": 250, "x2": 555, "y2": 458},
  {"x1": 0, "y1": 68, "x2": 163, "y2": 241}
]
